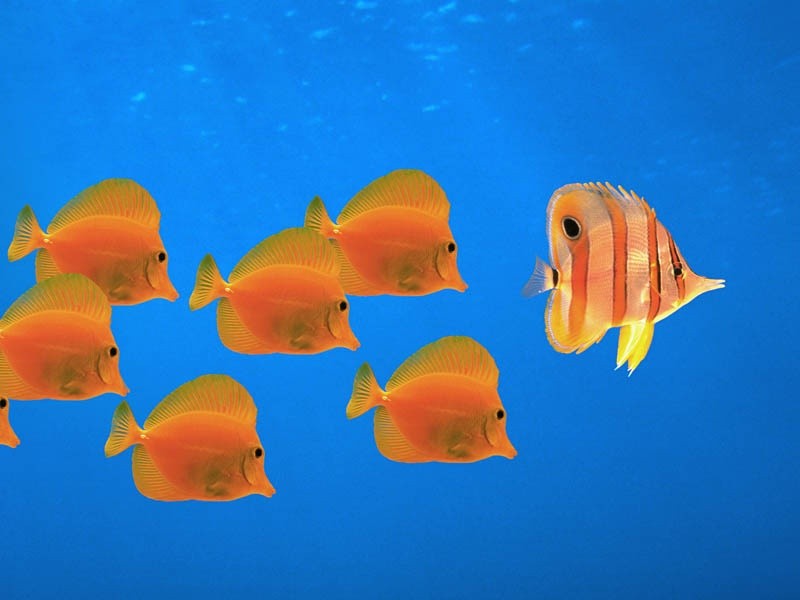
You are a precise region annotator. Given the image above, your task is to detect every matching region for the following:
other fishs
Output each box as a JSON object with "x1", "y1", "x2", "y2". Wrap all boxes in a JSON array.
[
  {"x1": 521, "y1": 182, "x2": 726, "y2": 377},
  {"x1": 0, "y1": 168, "x2": 517, "y2": 501}
]
